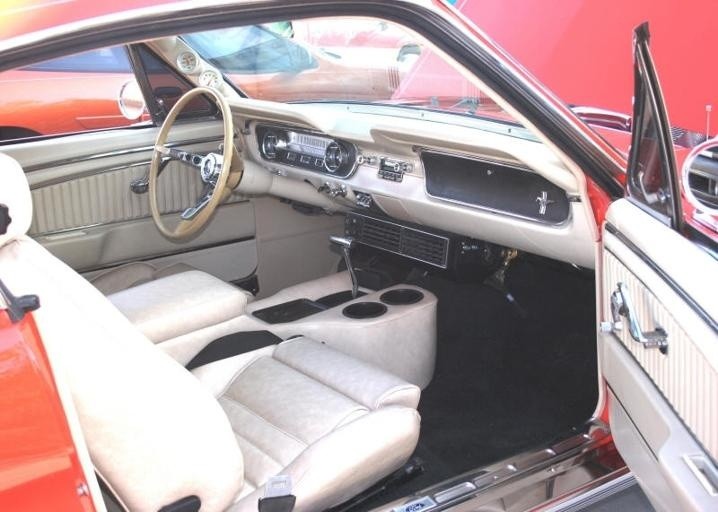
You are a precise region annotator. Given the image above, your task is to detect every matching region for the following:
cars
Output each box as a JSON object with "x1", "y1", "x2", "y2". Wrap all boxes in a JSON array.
[
  {"x1": 0, "y1": 0, "x2": 718, "y2": 511},
  {"x1": 0, "y1": 0, "x2": 408, "y2": 142}
]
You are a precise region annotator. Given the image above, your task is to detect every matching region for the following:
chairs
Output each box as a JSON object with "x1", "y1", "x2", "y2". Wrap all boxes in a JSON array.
[
  {"x1": 0, "y1": 153, "x2": 421, "y2": 512},
  {"x1": 93, "y1": 261, "x2": 251, "y2": 342}
]
